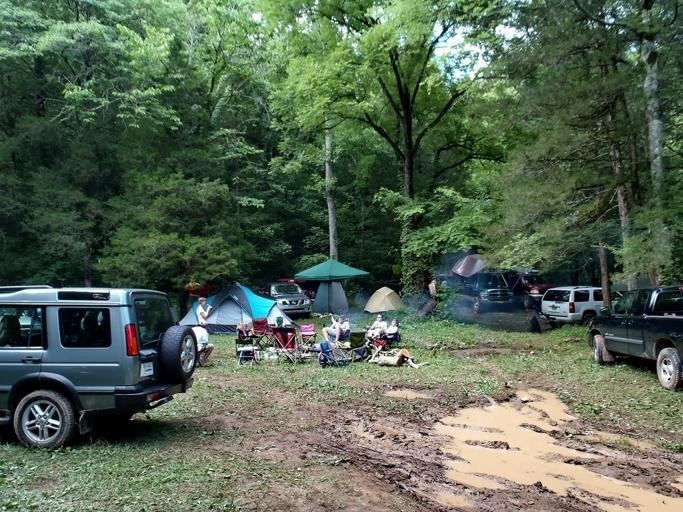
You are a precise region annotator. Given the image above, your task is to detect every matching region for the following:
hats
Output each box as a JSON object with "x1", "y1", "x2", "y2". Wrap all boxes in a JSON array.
[
  {"x1": 199, "y1": 297, "x2": 206, "y2": 301},
  {"x1": 199, "y1": 321, "x2": 206, "y2": 326}
]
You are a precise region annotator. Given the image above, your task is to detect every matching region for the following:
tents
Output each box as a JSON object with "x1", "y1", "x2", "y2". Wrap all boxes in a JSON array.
[
  {"x1": 363, "y1": 286, "x2": 406, "y2": 314},
  {"x1": 313, "y1": 280, "x2": 349, "y2": 315},
  {"x1": 179, "y1": 281, "x2": 298, "y2": 335}
]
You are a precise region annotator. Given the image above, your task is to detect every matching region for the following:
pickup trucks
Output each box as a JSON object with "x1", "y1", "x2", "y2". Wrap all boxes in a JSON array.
[
  {"x1": 436, "y1": 267, "x2": 551, "y2": 315},
  {"x1": 586, "y1": 284, "x2": 683, "y2": 392},
  {"x1": 258, "y1": 282, "x2": 312, "y2": 318}
]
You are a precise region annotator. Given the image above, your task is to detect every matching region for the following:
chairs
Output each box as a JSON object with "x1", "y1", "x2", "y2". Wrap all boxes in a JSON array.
[
  {"x1": 232, "y1": 316, "x2": 400, "y2": 368},
  {"x1": 1, "y1": 315, "x2": 23, "y2": 347}
]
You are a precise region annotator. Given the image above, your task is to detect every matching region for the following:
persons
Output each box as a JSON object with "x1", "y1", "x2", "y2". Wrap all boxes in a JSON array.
[
  {"x1": 373, "y1": 318, "x2": 399, "y2": 355},
  {"x1": 192, "y1": 323, "x2": 214, "y2": 367},
  {"x1": 197, "y1": 296, "x2": 213, "y2": 324},
  {"x1": 367, "y1": 314, "x2": 387, "y2": 341},
  {"x1": 322, "y1": 313, "x2": 349, "y2": 342}
]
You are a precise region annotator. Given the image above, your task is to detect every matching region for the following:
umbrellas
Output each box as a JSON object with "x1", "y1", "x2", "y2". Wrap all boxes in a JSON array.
[{"x1": 295, "y1": 258, "x2": 370, "y2": 313}]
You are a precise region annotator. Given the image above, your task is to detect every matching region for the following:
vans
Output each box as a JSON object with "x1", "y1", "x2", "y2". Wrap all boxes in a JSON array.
[{"x1": 540, "y1": 285, "x2": 625, "y2": 329}]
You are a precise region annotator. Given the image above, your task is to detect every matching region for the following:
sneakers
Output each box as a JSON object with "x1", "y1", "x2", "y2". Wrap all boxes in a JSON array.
[{"x1": 198, "y1": 359, "x2": 206, "y2": 367}]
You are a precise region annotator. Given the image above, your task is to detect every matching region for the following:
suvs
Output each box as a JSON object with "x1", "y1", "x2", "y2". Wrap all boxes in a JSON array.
[{"x1": 0, "y1": 284, "x2": 199, "y2": 453}]
